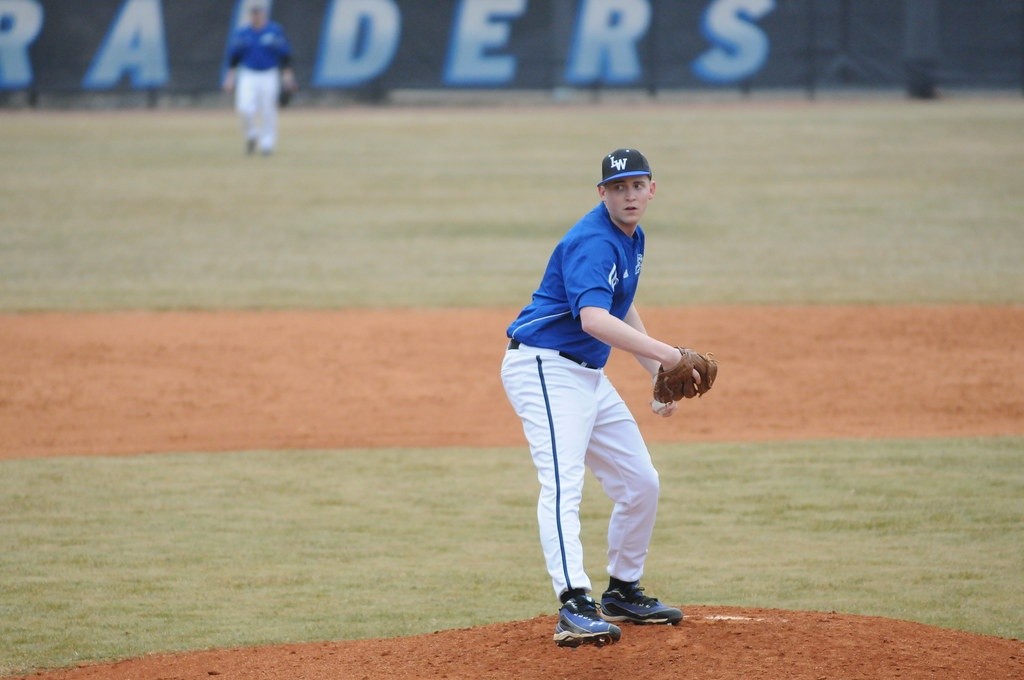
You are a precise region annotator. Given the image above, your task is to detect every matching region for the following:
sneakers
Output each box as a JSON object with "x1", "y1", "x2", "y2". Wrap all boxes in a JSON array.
[
  {"x1": 552, "y1": 595, "x2": 621, "y2": 648},
  {"x1": 600, "y1": 579, "x2": 683, "y2": 625}
]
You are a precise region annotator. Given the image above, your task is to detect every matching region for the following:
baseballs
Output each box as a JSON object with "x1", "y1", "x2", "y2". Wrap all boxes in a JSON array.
[{"x1": 652, "y1": 399, "x2": 671, "y2": 416}]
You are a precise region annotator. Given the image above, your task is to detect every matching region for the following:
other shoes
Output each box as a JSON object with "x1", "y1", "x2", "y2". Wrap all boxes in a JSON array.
[
  {"x1": 245, "y1": 136, "x2": 255, "y2": 151},
  {"x1": 261, "y1": 145, "x2": 273, "y2": 156}
]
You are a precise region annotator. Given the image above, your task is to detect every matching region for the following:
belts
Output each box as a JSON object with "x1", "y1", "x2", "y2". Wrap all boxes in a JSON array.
[{"x1": 507, "y1": 339, "x2": 600, "y2": 369}]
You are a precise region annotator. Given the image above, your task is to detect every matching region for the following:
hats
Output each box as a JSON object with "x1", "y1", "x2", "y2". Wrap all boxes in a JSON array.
[{"x1": 596, "y1": 148, "x2": 652, "y2": 187}]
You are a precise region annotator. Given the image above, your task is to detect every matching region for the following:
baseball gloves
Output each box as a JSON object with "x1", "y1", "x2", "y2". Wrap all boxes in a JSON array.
[
  {"x1": 652, "y1": 346, "x2": 720, "y2": 404},
  {"x1": 278, "y1": 86, "x2": 298, "y2": 106}
]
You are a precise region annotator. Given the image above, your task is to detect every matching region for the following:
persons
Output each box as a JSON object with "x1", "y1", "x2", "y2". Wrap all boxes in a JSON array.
[
  {"x1": 500, "y1": 148, "x2": 720, "y2": 648},
  {"x1": 223, "y1": 0, "x2": 293, "y2": 154}
]
